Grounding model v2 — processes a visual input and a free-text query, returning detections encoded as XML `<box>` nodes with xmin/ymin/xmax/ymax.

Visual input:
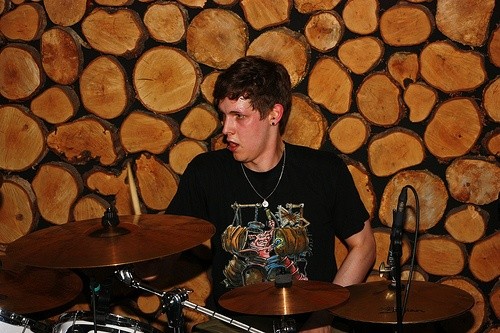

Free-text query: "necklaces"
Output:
<box><xmin>240</xmin><ymin>139</ymin><xmax>286</xmax><ymax>208</ymax></box>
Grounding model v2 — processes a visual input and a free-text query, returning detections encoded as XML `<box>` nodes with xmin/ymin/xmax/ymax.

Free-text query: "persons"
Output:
<box><xmin>165</xmin><ymin>56</ymin><xmax>377</xmax><ymax>333</ymax></box>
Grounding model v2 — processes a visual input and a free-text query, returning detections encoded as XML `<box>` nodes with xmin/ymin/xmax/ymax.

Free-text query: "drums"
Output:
<box><xmin>53</xmin><ymin>310</ymin><xmax>145</xmax><ymax>333</ymax></box>
<box><xmin>0</xmin><ymin>307</ymin><xmax>53</xmax><ymax>333</ymax></box>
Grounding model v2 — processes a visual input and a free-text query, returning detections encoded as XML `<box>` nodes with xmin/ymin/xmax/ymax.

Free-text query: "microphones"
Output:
<box><xmin>387</xmin><ymin>186</ymin><xmax>407</xmax><ymax>266</ymax></box>
<box><xmin>89</xmin><ymin>277</ymin><xmax>102</xmax><ymax>296</ymax></box>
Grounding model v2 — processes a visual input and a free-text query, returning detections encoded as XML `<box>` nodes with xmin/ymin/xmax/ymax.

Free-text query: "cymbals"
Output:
<box><xmin>328</xmin><ymin>280</ymin><xmax>475</xmax><ymax>324</ymax></box>
<box><xmin>5</xmin><ymin>215</ymin><xmax>217</xmax><ymax>269</ymax></box>
<box><xmin>0</xmin><ymin>255</ymin><xmax>83</xmax><ymax>315</ymax></box>
<box><xmin>219</xmin><ymin>280</ymin><xmax>350</xmax><ymax>315</ymax></box>
